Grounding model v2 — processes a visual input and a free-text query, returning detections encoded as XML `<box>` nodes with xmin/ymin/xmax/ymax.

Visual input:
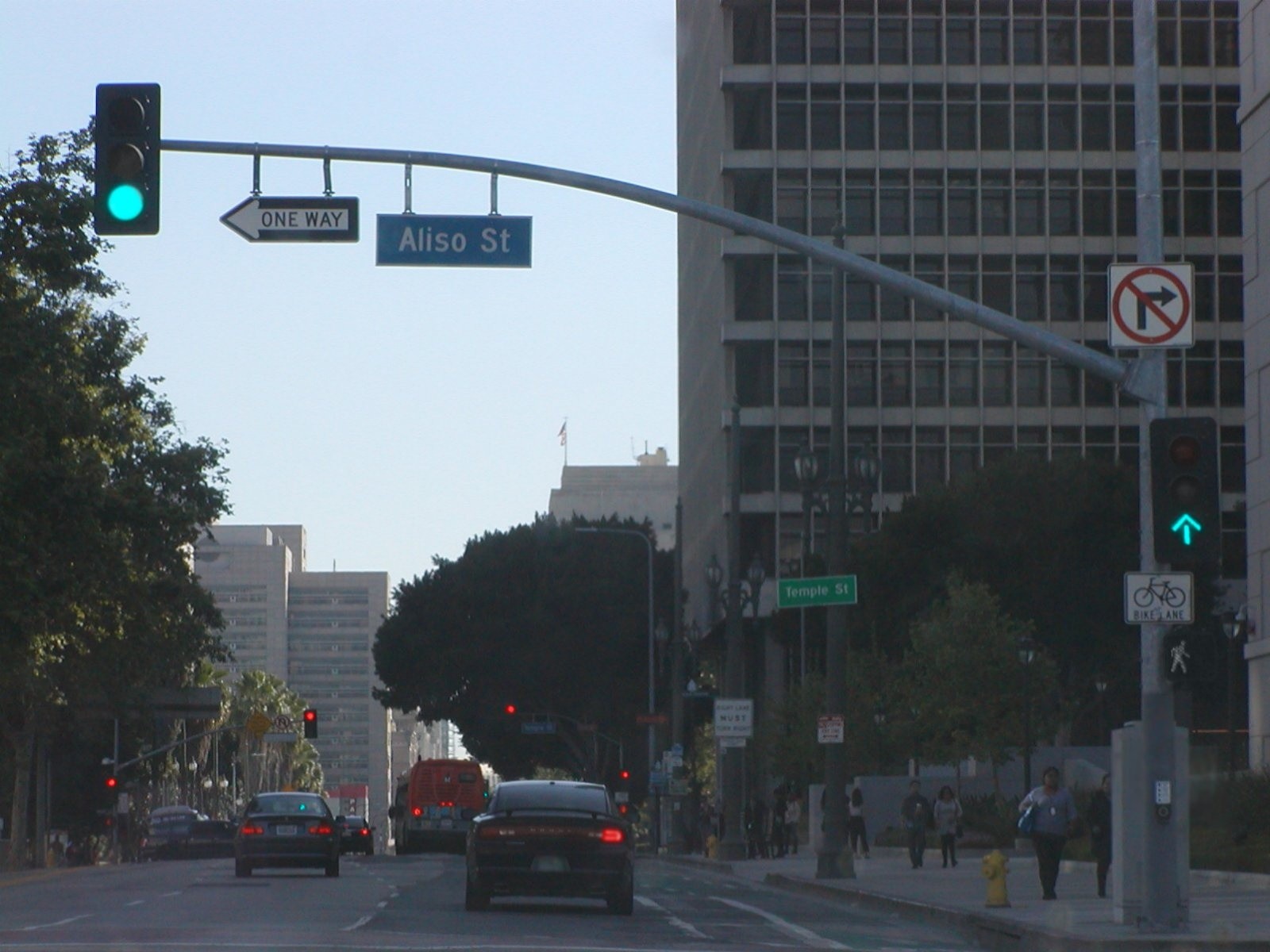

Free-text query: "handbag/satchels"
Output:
<box><xmin>1016</xmin><ymin>792</ymin><xmax>1040</xmax><ymax>833</ymax></box>
<box><xmin>956</xmin><ymin>823</ymin><xmax>963</xmax><ymax>838</ymax></box>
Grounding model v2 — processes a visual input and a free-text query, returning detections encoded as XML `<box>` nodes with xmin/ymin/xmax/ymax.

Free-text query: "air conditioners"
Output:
<box><xmin>331</xmin><ymin>622</ymin><xmax>338</xmax><ymax>627</ymax></box>
<box><xmin>332</xmin><ymin>758</ymin><xmax>339</xmax><ymax>764</ymax></box>
<box><xmin>332</xmin><ymin>645</ymin><xmax>338</xmax><ymax>650</ymax></box>
<box><xmin>331</xmin><ymin>715</ymin><xmax>339</xmax><ymax>721</ymax></box>
<box><xmin>229</xmin><ymin>667</ymin><xmax>236</xmax><ymax>673</ymax></box>
<box><xmin>229</xmin><ymin>619</ymin><xmax>236</xmax><ymax>626</ymax></box>
<box><xmin>230</xmin><ymin>643</ymin><xmax>237</xmax><ymax>649</ymax></box>
<box><xmin>331</xmin><ymin>692</ymin><xmax>338</xmax><ymax>698</ymax></box>
<box><xmin>229</xmin><ymin>690</ymin><xmax>237</xmax><ymax>696</ymax></box>
<box><xmin>332</xmin><ymin>598</ymin><xmax>338</xmax><ymax>603</ymax></box>
<box><xmin>331</xmin><ymin>785</ymin><xmax>339</xmax><ymax>791</ymax></box>
<box><xmin>331</xmin><ymin>738</ymin><xmax>338</xmax><ymax>743</ymax></box>
<box><xmin>230</xmin><ymin>596</ymin><xmax>236</xmax><ymax>602</ymax></box>
<box><xmin>332</xmin><ymin>668</ymin><xmax>338</xmax><ymax>674</ymax></box>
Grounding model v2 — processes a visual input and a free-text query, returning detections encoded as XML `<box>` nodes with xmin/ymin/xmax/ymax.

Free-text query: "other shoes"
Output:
<box><xmin>1042</xmin><ymin>890</ymin><xmax>1058</xmax><ymax>901</ymax></box>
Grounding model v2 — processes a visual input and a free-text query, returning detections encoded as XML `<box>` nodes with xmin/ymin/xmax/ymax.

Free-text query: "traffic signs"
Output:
<box><xmin>220</xmin><ymin>195</ymin><xmax>359</xmax><ymax>242</ymax></box>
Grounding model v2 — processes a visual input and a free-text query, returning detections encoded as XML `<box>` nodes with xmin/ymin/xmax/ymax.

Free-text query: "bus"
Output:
<box><xmin>388</xmin><ymin>755</ymin><xmax>490</xmax><ymax>857</ymax></box>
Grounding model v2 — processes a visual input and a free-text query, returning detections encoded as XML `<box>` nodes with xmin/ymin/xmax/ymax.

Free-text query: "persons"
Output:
<box><xmin>297</xmin><ymin>778</ymin><xmax>330</xmax><ymax>800</ymax></box>
<box><xmin>655</xmin><ymin>801</ymin><xmax>726</xmax><ymax>857</ymax></box>
<box><xmin>900</xmin><ymin>780</ymin><xmax>929</xmax><ymax>869</ymax></box>
<box><xmin>934</xmin><ymin>786</ymin><xmax>963</xmax><ymax>868</ymax></box>
<box><xmin>1089</xmin><ymin>773</ymin><xmax>1112</xmax><ymax>898</ymax></box>
<box><xmin>1019</xmin><ymin>766</ymin><xmax>1078</xmax><ymax>901</ymax></box>
<box><xmin>848</xmin><ymin>787</ymin><xmax>871</xmax><ymax>859</ymax></box>
<box><xmin>744</xmin><ymin>788</ymin><xmax>801</xmax><ymax>860</ymax></box>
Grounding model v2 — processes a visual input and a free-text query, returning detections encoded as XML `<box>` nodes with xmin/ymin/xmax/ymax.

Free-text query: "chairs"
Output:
<box><xmin>261</xmin><ymin>806</ymin><xmax>274</xmax><ymax>812</ymax></box>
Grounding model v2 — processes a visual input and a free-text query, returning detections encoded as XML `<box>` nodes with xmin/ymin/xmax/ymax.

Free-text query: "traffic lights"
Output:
<box><xmin>618</xmin><ymin>770</ymin><xmax>632</xmax><ymax>779</ymax></box>
<box><xmin>506</xmin><ymin>703</ymin><xmax>516</xmax><ymax>716</ymax></box>
<box><xmin>619</xmin><ymin>806</ymin><xmax>628</xmax><ymax>816</ymax></box>
<box><xmin>1164</xmin><ymin>626</ymin><xmax>1211</xmax><ymax>675</ymax></box>
<box><xmin>1149</xmin><ymin>419</ymin><xmax>1222</xmax><ymax>566</ymax></box>
<box><xmin>482</xmin><ymin>780</ymin><xmax>490</xmax><ymax>799</ymax></box>
<box><xmin>303</xmin><ymin>708</ymin><xmax>319</xmax><ymax>737</ymax></box>
<box><xmin>105</xmin><ymin>777</ymin><xmax>118</xmax><ymax>788</ymax></box>
<box><xmin>93</xmin><ymin>83</ymin><xmax>160</xmax><ymax>235</ymax></box>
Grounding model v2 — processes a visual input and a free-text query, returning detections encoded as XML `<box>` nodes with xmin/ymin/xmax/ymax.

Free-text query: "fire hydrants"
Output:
<box><xmin>705</xmin><ymin>834</ymin><xmax>721</xmax><ymax>860</ymax></box>
<box><xmin>981</xmin><ymin>850</ymin><xmax>1013</xmax><ymax>908</ymax></box>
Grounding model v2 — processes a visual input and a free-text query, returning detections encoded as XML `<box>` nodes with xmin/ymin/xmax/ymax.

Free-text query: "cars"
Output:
<box><xmin>235</xmin><ymin>791</ymin><xmax>341</xmax><ymax>878</ymax></box>
<box><xmin>146</xmin><ymin>804</ymin><xmax>241</xmax><ymax>857</ymax></box>
<box><xmin>336</xmin><ymin>815</ymin><xmax>378</xmax><ymax>856</ymax></box>
<box><xmin>466</xmin><ymin>781</ymin><xmax>635</xmax><ymax>917</ymax></box>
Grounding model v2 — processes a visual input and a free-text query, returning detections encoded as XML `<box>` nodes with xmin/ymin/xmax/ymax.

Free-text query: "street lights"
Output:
<box><xmin>104</xmin><ymin>757</ymin><xmax>121</xmax><ymax>865</ymax></box>
<box><xmin>574</xmin><ymin>526</ymin><xmax>660</xmax><ymax>856</ymax></box>
<box><xmin>203</xmin><ymin>773</ymin><xmax>229</xmax><ymax>822</ymax></box>
<box><xmin>702</xmin><ymin>391</ymin><xmax>766</xmax><ymax>864</ymax></box>
<box><xmin>173</xmin><ymin>756</ymin><xmax>197</xmax><ymax>805</ymax></box>
<box><xmin>796</xmin><ymin>203</ymin><xmax>878</xmax><ymax>883</ymax></box>
